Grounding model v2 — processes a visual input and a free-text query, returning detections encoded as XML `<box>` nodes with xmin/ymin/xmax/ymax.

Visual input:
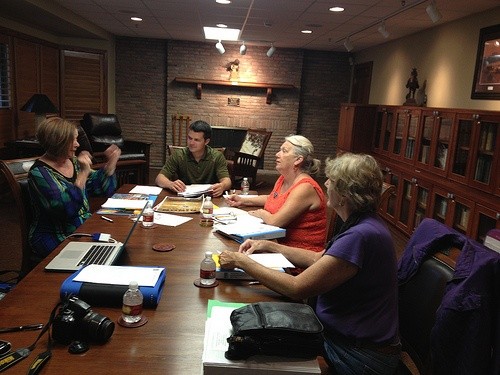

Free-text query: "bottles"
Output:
<box><xmin>200</xmin><ymin>251</ymin><xmax>216</xmax><ymax>286</ymax></box>
<box><xmin>142</xmin><ymin>203</ymin><xmax>154</xmax><ymax>227</ymax></box>
<box><xmin>203</xmin><ymin>197</ymin><xmax>213</xmax><ymax>218</ymax></box>
<box><xmin>241</xmin><ymin>178</ymin><xmax>250</xmax><ymax>195</ymax></box>
<box><xmin>121</xmin><ymin>281</ymin><xmax>144</xmax><ymax>323</ymax></box>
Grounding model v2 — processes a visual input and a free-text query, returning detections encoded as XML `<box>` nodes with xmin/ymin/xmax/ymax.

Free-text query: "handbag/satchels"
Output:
<box><xmin>225</xmin><ymin>301</ymin><xmax>324</xmax><ymax>361</ymax></box>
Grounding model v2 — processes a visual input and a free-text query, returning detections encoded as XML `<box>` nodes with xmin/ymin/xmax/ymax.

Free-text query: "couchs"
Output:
<box><xmin>81</xmin><ymin>114</ymin><xmax>152</xmax><ymax>186</ymax></box>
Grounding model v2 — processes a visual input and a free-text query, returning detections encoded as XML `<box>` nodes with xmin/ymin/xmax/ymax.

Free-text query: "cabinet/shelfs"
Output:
<box><xmin>337</xmin><ymin>102</ymin><xmax>500</xmax><ymax>250</ymax></box>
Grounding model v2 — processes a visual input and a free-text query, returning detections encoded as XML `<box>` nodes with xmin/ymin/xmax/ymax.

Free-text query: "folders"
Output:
<box><xmin>217</xmin><ymin>223</ymin><xmax>286</xmax><ymax>244</ymax></box>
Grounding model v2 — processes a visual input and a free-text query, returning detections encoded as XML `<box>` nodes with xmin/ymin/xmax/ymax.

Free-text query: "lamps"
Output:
<box><xmin>426</xmin><ymin>0</ymin><xmax>443</xmax><ymax>23</ymax></box>
<box><xmin>266</xmin><ymin>41</ymin><xmax>276</xmax><ymax>58</ymax></box>
<box><xmin>377</xmin><ymin>20</ymin><xmax>391</xmax><ymax>39</ymax></box>
<box><xmin>215</xmin><ymin>40</ymin><xmax>226</xmax><ymax>55</ymax></box>
<box><xmin>239</xmin><ymin>41</ymin><xmax>247</xmax><ymax>55</ymax></box>
<box><xmin>343</xmin><ymin>37</ymin><xmax>354</xmax><ymax>52</ymax></box>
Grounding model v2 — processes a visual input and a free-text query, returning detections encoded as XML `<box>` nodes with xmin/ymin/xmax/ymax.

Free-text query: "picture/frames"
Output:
<box><xmin>471</xmin><ymin>25</ymin><xmax>500</xmax><ymax>100</ymax></box>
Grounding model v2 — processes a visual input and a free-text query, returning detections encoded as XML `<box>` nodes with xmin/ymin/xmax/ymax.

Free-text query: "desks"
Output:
<box><xmin>0</xmin><ymin>183</ymin><xmax>322</xmax><ymax>375</ymax></box>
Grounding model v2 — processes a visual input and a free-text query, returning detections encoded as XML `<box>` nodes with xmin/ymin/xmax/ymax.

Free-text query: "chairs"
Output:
<box><xmin>223</xmin><ymin>129</ymin><xmax>272</xmax><ymax>188</ymax></box>
<box><xmin>0</xmin><ymin>155</ymin><xmax>41</xmax><ymax>283</ymax></box>
<box><xmin>168</xmin><ymin>145</ymin><xmax>227</xmax><ymax>156</ymax></box>
<box><xmin>392</xmin><ymin>218</ymin><xmax>500</xmax><ymax>375</ymax></box>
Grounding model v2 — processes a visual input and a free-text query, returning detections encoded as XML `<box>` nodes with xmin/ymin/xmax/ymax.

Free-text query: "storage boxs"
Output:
<box><xmin>202</xmin><ymin>298</ymin><xmax>321</xmax><ymax>375</ymax></box>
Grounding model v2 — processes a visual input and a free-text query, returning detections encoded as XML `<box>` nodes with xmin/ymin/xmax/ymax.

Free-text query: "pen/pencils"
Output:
<box><xmin>233</xmin><ymin>188</ymin><xmax>235</xmax><ymax>193</ymax></box>
<box><xmin>225</xmin><ymin>191</ymin><xmax>229</xmax><ymax>199</ymax></box>
<box><xmin>100</xmin><ymin>215</ymin><xmax>113</xmax><ymax>221</ymax></box>
<box><xmin>249</xmin><ymin>282</ymin><xmax>260</xmax><ymax>284</ymax></box>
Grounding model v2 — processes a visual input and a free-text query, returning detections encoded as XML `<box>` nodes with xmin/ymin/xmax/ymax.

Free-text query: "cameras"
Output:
<box><xmin>52</xmin><ymin>292</ymin><xmax>115</xmax><ymax>344</ymax></box>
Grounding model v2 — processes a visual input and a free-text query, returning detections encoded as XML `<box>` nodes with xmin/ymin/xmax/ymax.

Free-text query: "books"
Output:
<box><xmin>406</xmin><ymin>125</ymin><xmax>493</xmax><ymax>228</ymax></box>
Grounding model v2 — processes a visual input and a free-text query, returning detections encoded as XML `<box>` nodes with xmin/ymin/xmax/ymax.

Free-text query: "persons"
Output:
<box><xmin>226</xmin><ymin>135</ymin><xmax>327</xmax><ymax>275</ymax></box>
<box><xmin>27</xmin><ymin>117</ymin><xmax>121</xmax><ymax>256</ymax></box>
<box><xmin>218</xmin><ymin>151</ymin><xmax>403</xmax><ymax>375</ymax></box>
<box><xmin>406</xmin><ymin>68</ymin><xmax>419</xmax><ymax>98</ymax></box>
<box><xmin>155</xmin><ymin>120</ymin><xmax>231</xmax><ymax>197</ymax></box>
